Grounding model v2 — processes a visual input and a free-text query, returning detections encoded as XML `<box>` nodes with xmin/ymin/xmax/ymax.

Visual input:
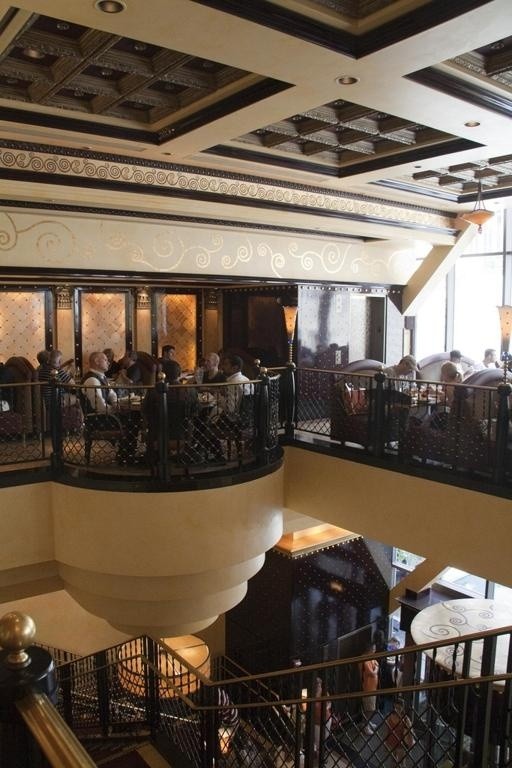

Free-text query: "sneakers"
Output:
<box><xmin>360</xmin><ymin>721</ymin><xmax>377</xmax><ymax>736</ymax></box>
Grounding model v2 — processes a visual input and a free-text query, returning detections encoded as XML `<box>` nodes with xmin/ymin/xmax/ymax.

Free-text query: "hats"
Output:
<box><xmin>403</xmin><ymin>355</ymin><xmax>421</xmax><ymax>374</ymax></box>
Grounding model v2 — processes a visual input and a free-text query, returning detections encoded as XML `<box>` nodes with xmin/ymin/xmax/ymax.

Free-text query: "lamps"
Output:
<box><xmin>282</xmin><ymin>305</ymin><xmax>302</xmax><ymax>362</ymax></box>
<box><xmin>461</xmin><ymin>164</ymin><xmax>495</xmax><ymax>235</ymax></box>
<box><xmin>495</xmin><ymin>304</ymin><xmax>511</xmax><ymax>384</ymax></box>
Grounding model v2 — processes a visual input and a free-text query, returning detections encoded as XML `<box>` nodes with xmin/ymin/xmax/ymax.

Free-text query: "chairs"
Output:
<box><xmin>0</xmin><ymin>343</ymin><xmax>511</xmax><ymax>497</ymax></box>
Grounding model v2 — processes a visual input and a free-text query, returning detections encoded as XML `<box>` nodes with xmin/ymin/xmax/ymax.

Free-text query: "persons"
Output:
<box><xmin>470</xmin><ymin>349</ymin><xmax>499</xmax><ymax>375</ymax></box>
<box><xmin>372</xmin><ymin>355</ymin><xmax>421</xmax><ymax>399</ymax></box>
<box><xmin>313</xmin><ymin>677</ymin><xmax>333</xmax><ymax>768</ymax></box>
<box><xmin>387</xmin><ymin>636</ymin><xmax>401</xmax><ymax>651</ymax></box>
<box><xmin>1</xmin><ymin>360</ymin><xmax>19</xmax><ymax>415</ymax></box>
<box><xmin>38</xmin><ymin>343</ymin><xmax>276</xmax><ymax>468</ymax></box>
<box><xmin>423</xmin><ymin>361</ymin><xmax>463</xmax><ymax>433</ymax></box>
<box><xmin>384</xmin><ymin>698</ymin><xmax>414</xmax><ymax>768</ymax></box>
<box><xmin>216</xmin><ymin>688</ymin><xmax>239</xmax><ymax>754</ymax></box>
<box><xmin>357</xmin><ymin>641</ymin><xmax>380</xmax><ymax>737</ymax></box>
<box><xmin>443</xmin><ymin>351</ymin><xmax>473</xmax><ymax>382</ymax></box>
